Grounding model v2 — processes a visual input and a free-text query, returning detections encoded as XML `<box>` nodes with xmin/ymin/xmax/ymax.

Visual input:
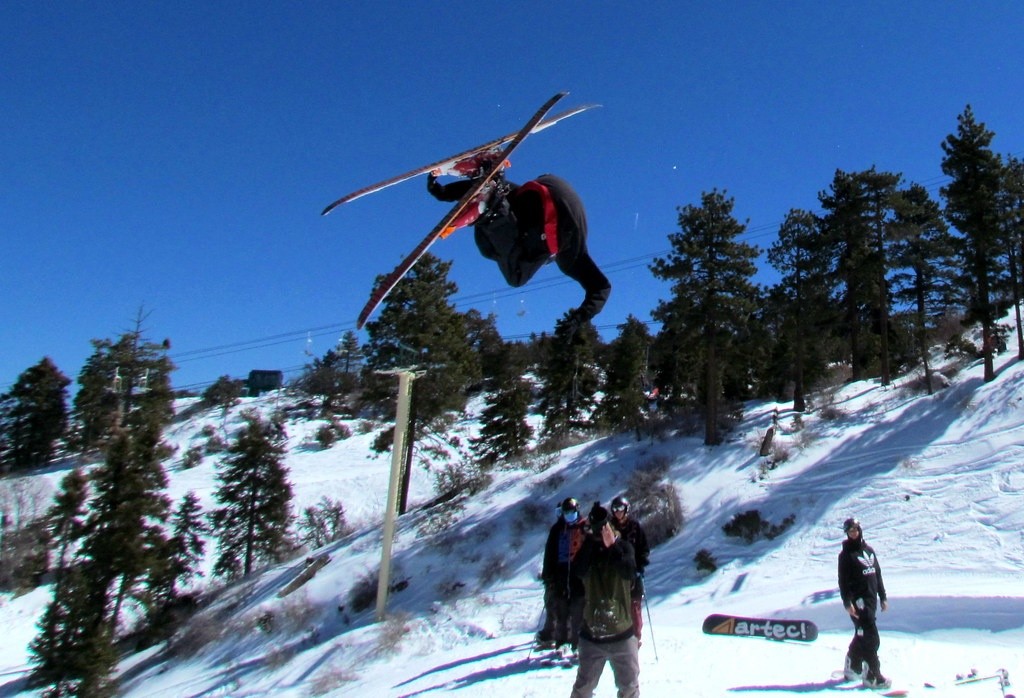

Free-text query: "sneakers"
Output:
<box><xmin>862</xmin><ymin>671</ymin><xmax>892</xmax><ymax>689</ymax></box>
<box><xmin>432</xmin><ymin>145</ymin><xmax>511</xmax><ymax>178</ymax></box>
<box><xmin>844</xmin><ymin>661</ymin><xmax>864</xmax><ymax>681</ymax></box>
<box><xmin>440</xmin><ymin>179</ymin><xmax>510</xmax><ymax>241</ymax></box>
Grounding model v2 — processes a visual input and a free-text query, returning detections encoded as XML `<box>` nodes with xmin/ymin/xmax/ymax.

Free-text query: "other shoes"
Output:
<box><xmin>536</xmin><ymin>629</ymin><xmax>554</xmax><ymax>645</ymax></box>
<box><xmin>557</xmin><ymin>648</ymin><xmax>578</xmax><ymax>658</ymax></box>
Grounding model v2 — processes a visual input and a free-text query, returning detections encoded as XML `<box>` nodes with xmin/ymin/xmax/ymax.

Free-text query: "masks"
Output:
<box><xmin>563</xmin><ymin>512</ymin><xmax>578</xmax><ymax>522</ymax></box>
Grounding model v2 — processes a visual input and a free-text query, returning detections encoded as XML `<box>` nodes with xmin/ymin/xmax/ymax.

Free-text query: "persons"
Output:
<box><xmin>537</xmin><ymin>497</ymin><xmax>584</xmax><ymax>656</ymax></box>
<box><xmin>428</xmin><ymin>172</ymin><xmax>610</xmax><ymax>337</ymax></box>
<box><xmin>570</xmin><ymin>501</ymin><xmax>640</xmax><ymax>698</ymax></box>
<box><xmin>611</xmin><ymin>496</ymin><xmax>650</xmax><ymax>646</ymax></box>
<box><xmin>838</xmin><ymin>518</ymin><xmax>892</xmax><ymax>690</ymax></box>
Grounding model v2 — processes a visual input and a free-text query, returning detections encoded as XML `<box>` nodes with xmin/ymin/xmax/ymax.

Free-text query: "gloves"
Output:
<box><xmin>427</xmin><ymin>173</ymin><xmax>437</xmax><ymax>192</ymax></box>
<box><xmin>555</xmin><ymin>317</ymin><xmax>580</xmax><ymax>343</ymax></box>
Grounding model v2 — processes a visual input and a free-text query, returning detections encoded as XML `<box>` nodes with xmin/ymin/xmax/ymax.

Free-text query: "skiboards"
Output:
<box><xmin>542</xmin><ymin>647</ymin><xmax>580</xmax><ymax>668</ymax></box>
<box><xmin>320</xmin><ymin>88</ymin><xmax>605</xmax><ymax>329</ymax></box>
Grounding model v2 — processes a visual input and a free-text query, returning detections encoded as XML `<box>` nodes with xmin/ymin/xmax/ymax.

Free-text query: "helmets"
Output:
<box><xmin>843</xmin><ymin>518</ymin><xmax>861</xmax><ymax>532</ymax></box>
<box><xmin>612</xmin><ymin>497</ymin><xmax>631</xmax><ymax>515</ymax></box>
<box><xmin>561</xmin><ymin>497</ymin><xmax>579</xmax><ymax>510</ymax></box>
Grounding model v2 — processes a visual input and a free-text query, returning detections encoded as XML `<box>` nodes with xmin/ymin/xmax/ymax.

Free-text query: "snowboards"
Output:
<box><xmin>701</xmin><ymin>612</ymin><xmax>819</xmax><ymax>643</ymax></box>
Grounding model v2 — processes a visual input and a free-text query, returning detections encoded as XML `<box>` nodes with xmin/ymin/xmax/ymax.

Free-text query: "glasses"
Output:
<box><xmin>611</xmin><ymin>505</ymin><xmax>627</xmax><ymax>512</ymax></box>
<box><xmin>563</xmin><ymin>507</ymin><xmax>577</xmax><ymax>514</ymax></box>
